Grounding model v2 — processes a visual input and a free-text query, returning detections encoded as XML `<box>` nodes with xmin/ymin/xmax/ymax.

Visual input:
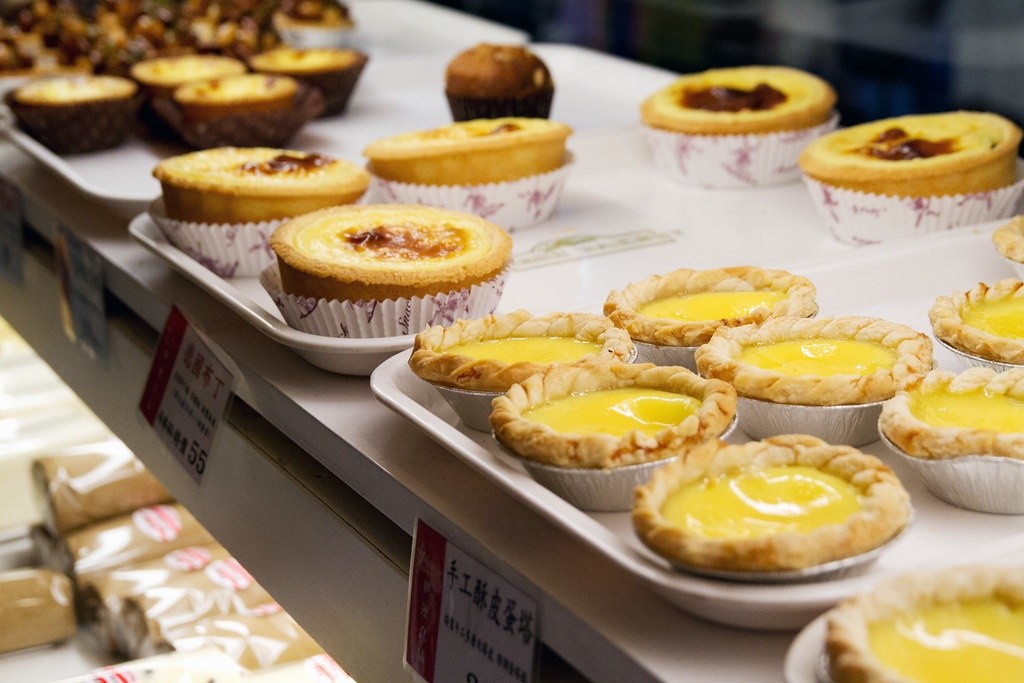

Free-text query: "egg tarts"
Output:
<box><xmin>406</xmin><ymin>217</ymin><xmax>1024</xmax><ymax>683</ymax></box>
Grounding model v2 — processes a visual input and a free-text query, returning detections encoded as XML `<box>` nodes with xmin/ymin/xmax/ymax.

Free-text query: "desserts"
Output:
<box><xmin>0</xmin><ymin>0</ymin><xmax>1024</xmax><ymax>338</ymax></box>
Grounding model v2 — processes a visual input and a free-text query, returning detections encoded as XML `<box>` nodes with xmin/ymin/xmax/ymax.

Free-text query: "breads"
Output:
<box><xmin>0</xmin><ymin>433</ymin><xmax>355</xmax><ymax>683</ymax></box>
